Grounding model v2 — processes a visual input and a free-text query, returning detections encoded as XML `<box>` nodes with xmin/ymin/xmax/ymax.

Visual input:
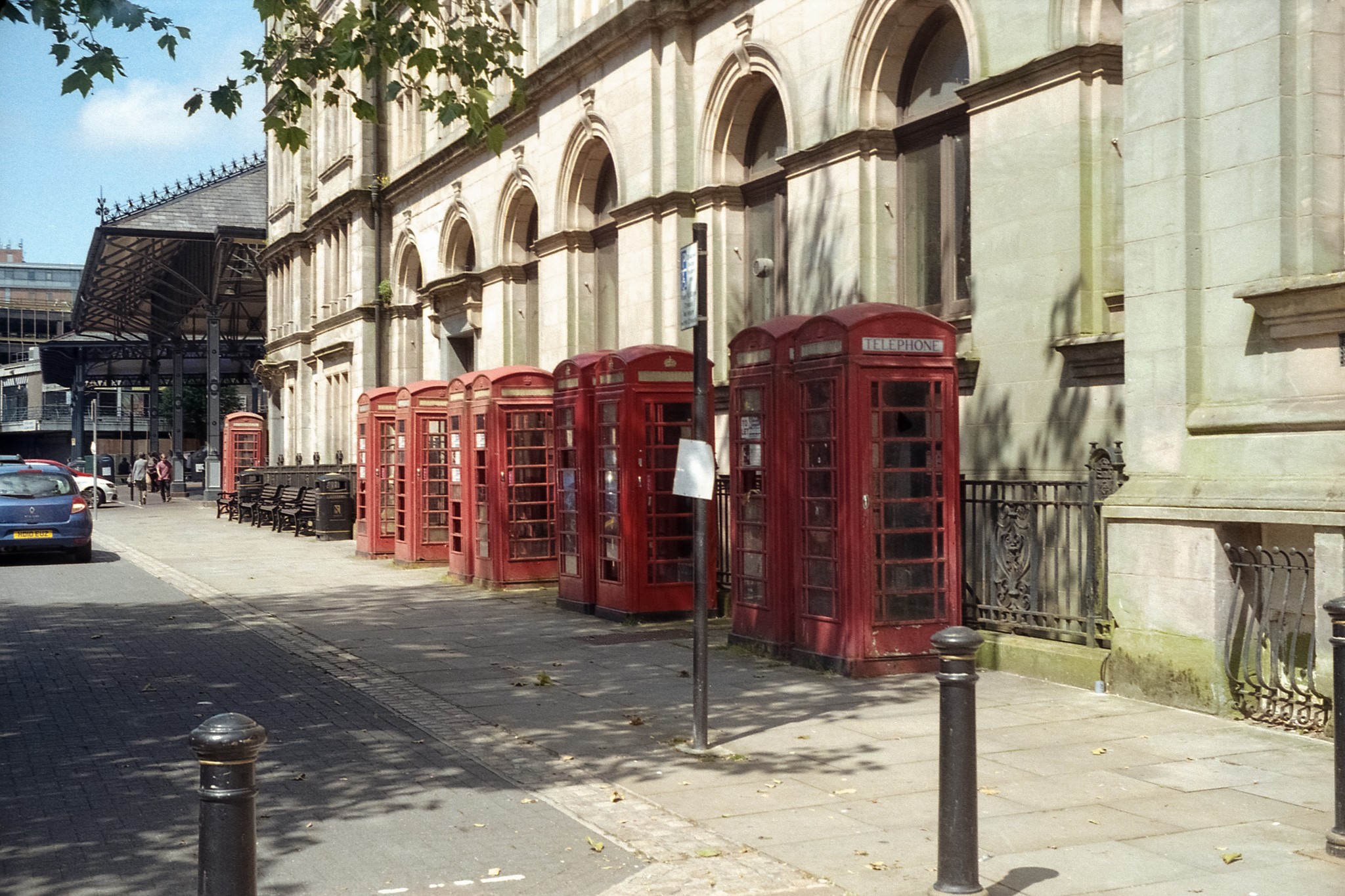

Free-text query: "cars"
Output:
<box><xmin>0</xmin><ymin>465</ymin><xmax>92</xmax><ymax>563</ymax></box>
<box><xmin>0</xmin><ymin>454</ymin><xmax>119</xmax><ymax>509</ymax></box>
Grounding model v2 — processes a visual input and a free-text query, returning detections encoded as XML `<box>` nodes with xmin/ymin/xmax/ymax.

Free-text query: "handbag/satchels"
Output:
<box><xmin>126</xmin><ymin>473</ymin><xmax>132</xmax><ymax>483</ymax></box>
<box><xmin>145</xmin><ymin>473</ymin><xmax>152</xmax><ymax>484</ymax></box>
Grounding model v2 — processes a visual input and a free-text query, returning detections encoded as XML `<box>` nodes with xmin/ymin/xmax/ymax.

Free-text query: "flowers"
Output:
<box><xmin>374</xmin><ymin>171</ymin><xmax>392</xmax><ymax>190</ymax></box>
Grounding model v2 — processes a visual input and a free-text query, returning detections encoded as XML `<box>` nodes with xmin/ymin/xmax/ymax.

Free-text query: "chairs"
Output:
<box><xmin>215</xmin><ymin>481</ymin><xmax>320</xmax><ymax>539</ymax></box>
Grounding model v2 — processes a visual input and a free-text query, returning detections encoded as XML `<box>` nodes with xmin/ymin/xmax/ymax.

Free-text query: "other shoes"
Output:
<box><xmin>142</xmin><ymin>496</ymin><xmax>146</xmax><ymax>504</ymax></box>
<box><xmin>166</xmin><ymin>496</ymin><xmax>171</xmax><ymax>502</ymax></box>
<box><xmin>138</xmin><ymin>502</ymin><xmax>143</xmax><ymax>506</ymax></box>
<box><xmin>162</xmin><ymin>499</ymin><xmax>165</xmax><ymax>503</ymax></box>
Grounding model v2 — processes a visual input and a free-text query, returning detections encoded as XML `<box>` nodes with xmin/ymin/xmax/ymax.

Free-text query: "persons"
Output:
<box><xmin>131</xmin><ymin>451</ymin><xmax>148</xmax><ymax>505</ymax></box>
<box><xmin>118</xmin><ymin>457</ymin><xmax>131</xmax><ymax>484</ymax></box>
<box><xmin>166</xmin><ymin>450</ymin><xmax>173</xmax><ymax>464</ymax></box>
<box><xmin>155</xmin><ymin>454</ymin><xmax>173</xmax><ymax>502</ymax></box>
<box><xmin>146</xmin><ymin>453</ymin><xmax>160</xmax><ymax>493</ymax></box>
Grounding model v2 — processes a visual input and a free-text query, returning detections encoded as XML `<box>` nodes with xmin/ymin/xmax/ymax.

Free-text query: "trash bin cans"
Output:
<box><xmin>238</xmin><ymin>469</ymin><xmax>263</xmax><ymax>518</ymax></box>
<box><xmin>314</xmin><ymin>472</ymin><xmax>352</xmax><ymax>541</ymax></box>
